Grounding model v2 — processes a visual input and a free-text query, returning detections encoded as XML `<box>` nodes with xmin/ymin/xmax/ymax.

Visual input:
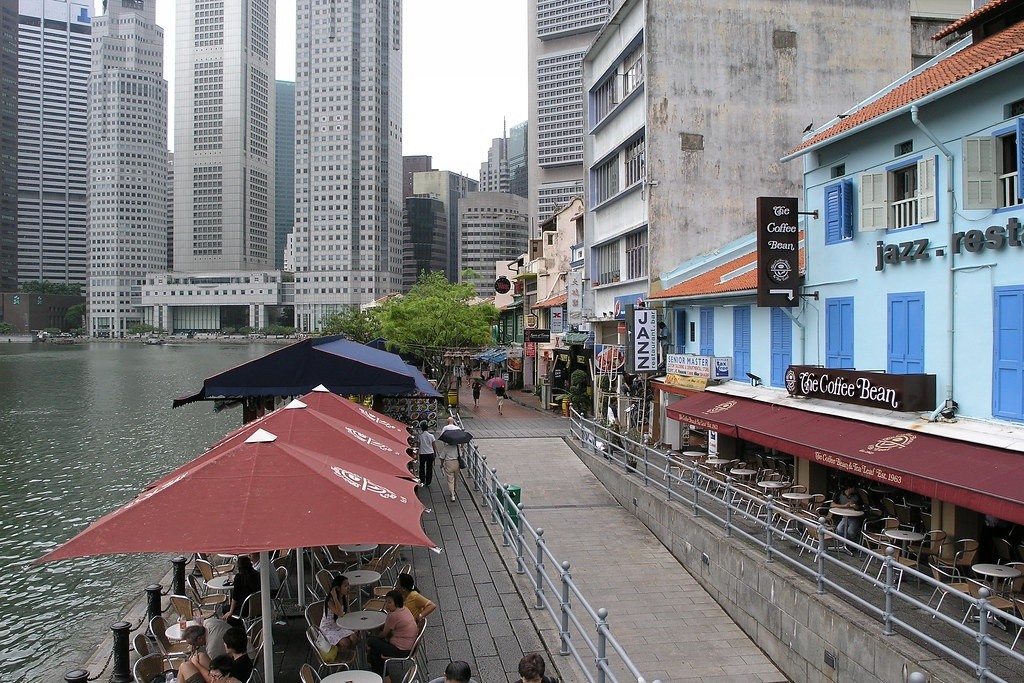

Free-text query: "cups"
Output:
<box><xmin>180</xmin><ymin>620</ymin><xmax>186</xmax><ymax>631</ymax></box>
<box><xmin>166</xmin><ymin>671</ymin><xmax>174</xmax><ymax>683</ymax></box>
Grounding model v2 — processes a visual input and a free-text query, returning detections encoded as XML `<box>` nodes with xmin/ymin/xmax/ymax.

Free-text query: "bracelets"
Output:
<box><xmin>419</xmin><ymin>614</ymin><xmax>422</xmax><ymax>617</ymax></box>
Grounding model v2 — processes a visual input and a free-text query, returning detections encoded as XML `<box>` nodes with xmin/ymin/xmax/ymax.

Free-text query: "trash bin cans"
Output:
<box><xmin>497</xmin><ymin>485</ymin><xmax>521</xmax><ymax>529</ymax></box>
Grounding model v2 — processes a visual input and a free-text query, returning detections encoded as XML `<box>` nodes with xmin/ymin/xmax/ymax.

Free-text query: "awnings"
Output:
<box><xmin>668</xmin><ymin>388</ymin><xmax>1024</xmax><ymax>526</ymax></box>
<box><xmin>473</xmin><ymin>348</ymin><xmax>506</xmax><ymax>366</ymax></box>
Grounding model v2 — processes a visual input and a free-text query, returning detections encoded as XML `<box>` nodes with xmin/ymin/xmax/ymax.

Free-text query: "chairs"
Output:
<box><xmin>663</xmin><ymin>450</ymin><xmax>1024</xmax><ymax>651</ymax></box>
<box><xmin>298</xmin><ymin>543</ymin><xmax>428</xmax><ymax>683</ymax></box>
<box><xmin>132</xmin><ymin>544</ymin><xmax>292</xmax><ymax>683</ymax></box>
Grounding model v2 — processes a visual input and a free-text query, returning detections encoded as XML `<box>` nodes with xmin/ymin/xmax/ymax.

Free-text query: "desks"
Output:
<box><xmin>320</xmin><ymin>670</ymin><xmax>383</xmax><ymax>683</ymax></box>
<box><xmin>217</xmin><ymin>553</ymin><xmax>250</xmax><ymax>573</ymax></box>
<box><xmin>705</xmin><ymin>459</ymin><xmax>730</xmax><ymax>492</ymax></box>
<box><xmin>758</xmin><ymin>481</ymin><xmax>785</xmax><ymax>521</ymax></box>
<box><xmin>165</xmin><ymin>621</ymin><xmax>199</xmax><ymax>655</ymax></box>
<box><xmin>781</xmin><ymin>493</ymin><xmax>813</xmax><ymax>536</ymax></box>
<box><xmin>342</xmin><ymin>570</ymin><xmax>381</xmax><ymax>611</ymax></box>
<box><xmin>730</xmin><ymin>468</ymin><xmax>757</xmax><ymax>503</ymax></box>
<box><xmin>683</xmin><ymin>451</ymin><xmax>706</xmax><ymax>483</ymax></box>
<box><xmin>829</xmin><ymin>507</ymin><xmax>865</xmax><ymax>556</ymax></box>
<box><xmin>884</xmin><ymin>530</ymin><xmax>924</xmax><ymax>583</ymax></box>
<box><xmin>336</xmin><ymin>610</ymin><xmax>387</xmax><ymax>670</ymax></box>
<box><xmin>338</xmin><ymin>544</ymin><xmax>378</xmax><ymax>597</ymax></box>
<box><xmin>969</xmin><ymin>563</ymin><xmax>1021</xmax><ymax>631</ymax></box>
<box><xmin>206</xmin><ymin>575</ymin><xmax>236</xmax><ymax>605</ymax></box>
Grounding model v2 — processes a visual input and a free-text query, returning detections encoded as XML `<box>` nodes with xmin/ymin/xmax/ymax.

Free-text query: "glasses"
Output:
<box><xmin>208</xmin><ymin>674</ymin><xmax>223</xmax><ymax>680</ymax></box>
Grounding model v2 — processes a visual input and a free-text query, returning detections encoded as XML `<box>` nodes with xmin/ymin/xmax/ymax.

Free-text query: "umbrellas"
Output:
<box><xmin>35</xmin><ymin>428</ymin><xmax>438</xmax><ymax>683</ymax></box>
<box><xmin>208</xmin><ymin>382</ymin><xmax>416</xmax><ymax>448</ymax></box>
<box><xmin>438</xmin><ymin>430</ymin><xmax>473</xmax><ymax>445</ymax></box>
<box><xmin>488</xmin><ymin>376</ymin><xmax>505</xmax><ymax>389</ymax></box>
<box><xmin>138</xmin><ymin>397</ymin><xmax>418</xmax><ymax>607</ymax></box>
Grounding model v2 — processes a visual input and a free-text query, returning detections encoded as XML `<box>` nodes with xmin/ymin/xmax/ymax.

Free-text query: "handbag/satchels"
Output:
<box><xmin>458</xmin><ymin>456</ymin><xmax>467</xmax><ymax>469</ymax></box>
<box><xmin>311</xmin><ymin>626</ymin><xmax>338</xmax><ymax>662</ymax></box>
<box><xmin>503</xmin><ymin>394</ymin><xmax>508</xmax><ymax>399</ymax></box>
<box><xmin>147</xmin><ymin>669</ymin><xmax>178</xmax><ymax>683</ymax></box>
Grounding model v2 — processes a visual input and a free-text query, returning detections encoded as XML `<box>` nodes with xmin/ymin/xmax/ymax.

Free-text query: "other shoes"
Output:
<box><xmin>451</xmin><ymin>496</ymin><xmax>455</xmax><ymax>502</ymax></box>
<box><xmin>426</xmin><ymin>484</ymin><xmax>431</xmax><ymax>487</ymax></box>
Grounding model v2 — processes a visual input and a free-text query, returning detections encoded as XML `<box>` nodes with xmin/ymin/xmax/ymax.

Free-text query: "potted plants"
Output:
<box><xmin>625</xmin><ymin>428</ymin><xmax>640</xmax><ymax>472</ymax></box>
<box><xmin>567</xmin><ymin>369</ymin><xmax>590</xmax><ymax>418</ymax></box>
<box><xmin>600</xmin><ymin>418</ymin><xmax>616</xmax><ymax>459</ymax></box>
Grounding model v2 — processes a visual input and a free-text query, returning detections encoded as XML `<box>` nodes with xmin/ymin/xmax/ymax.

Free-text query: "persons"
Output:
<box><xmin>439</xmin><ymin>443</ymin><xmax>461</xmax><ymax>501</ymax></box>
<box><xmin>174</xmin><ymin>553</ymin><xmax>280</xmax><ymax>683</ymax></box>
<box><xmin>366</xmin><ymin>591</ymin><xmax>418</xmax><ymax>683</ymax></box>
<box><xmin>496</xmin><ymin>385</ymin><xmax>505</xmax><ymax>415</ymax></box>
<box><xmin>396</xmin><ymin>573</ymin><xmax>436</xmax><ymax>623</ymax></box>
<box><xmin>430</xmin><ymin>661</ymin><xmax>476</xmax><ymax>683</ymax></box>
<box><xmin>513</xmin><ymin>653</ymin><xmax>558</xmax><ymax>683</ymax></box>
<box><xmin>317</xmin><ymin>575</ymin><xmax>359</xmax><ymax>649</ymax></box>
<box><xmin>441</xmin><ymin>417</ymin><xmax>462</xmax><ymax>434</ymax></box>
<box><xmin>457</xmin><ymin>362</ymin><xmax>471</xmax><ymax>387</ymax></box>
<box><xmin>830</xmin><ymin>480</ymin><xmax>863</xmax><ymax>551</ymax></box>
<box><xmin>472</xmin><ymin>379</ymin><xmax>482</xmax><ymax>407</ymax></box>
<box><xmin>418</xmin><ymin>423</ymin><xmax>439</xmax><ymax>486</ymax></box>
